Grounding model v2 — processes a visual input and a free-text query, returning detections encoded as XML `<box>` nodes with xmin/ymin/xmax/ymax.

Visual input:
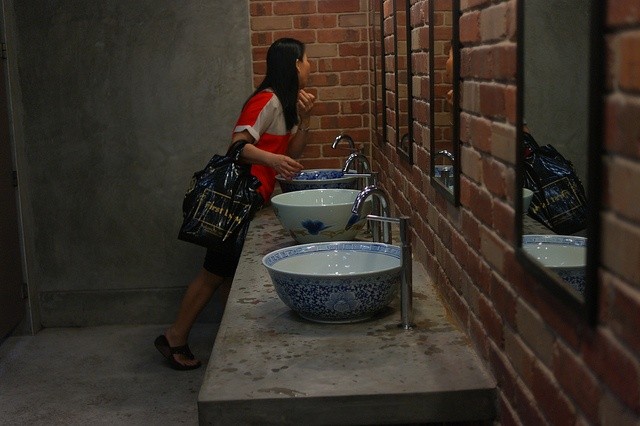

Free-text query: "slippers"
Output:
<box><xmin>154</xmin><ymin>335</ymin><xmax>201</xmax><ymax>371</ymax></box>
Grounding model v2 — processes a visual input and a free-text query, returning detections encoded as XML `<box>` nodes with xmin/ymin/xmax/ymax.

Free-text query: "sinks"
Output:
<box><xmin>434</xmin><ymin>164</ymin><xmax>454</xmax><ymax>179</ymax></box>
<box><xmin>272</xmin><ymin>188</ymin><xmax>372</xmax><ymax>242</ymax></box>
<box><xmin>263</xmin><ymin>241</ymin><xmax>413</xmax><ymax>323</ymax></box>
<box><xmin>276</xmin><ymin>168</ymin><xmax>358</xmax><ymax>192</ymax></box>
<box><xmin>523</xmin><ymin>187</ymin><xmax>533</xmax><ymax>199</ymax></box>
<box><xmin>522</xmin><ymin>234</ymin><xmax>587</xmax><ymax>267</ymax></box>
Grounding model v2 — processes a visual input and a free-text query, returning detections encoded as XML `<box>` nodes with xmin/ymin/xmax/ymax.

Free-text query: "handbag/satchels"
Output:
<box><xmin>176</xmin><ymin>138</ymin><xmax>264</xmax><ymax>250</ymax></box>
<box><xmin>520</xmin><ymin>132</ymin><xmax>587</xmax><ymax>235</ymax></box>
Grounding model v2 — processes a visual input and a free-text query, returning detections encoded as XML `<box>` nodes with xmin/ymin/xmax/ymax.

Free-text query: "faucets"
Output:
<box><xmin>350</xmin><ymin>184</ymin><xmax>393</xmax><ymax>244</ymax></box>
<box><xmin>343</xmin><ymin>151</ymin><xmax>371</xmax><ymax>188</ymax></box>
<box><xmin>433</xmin><ymin>149</ymin><xmax>454</xmax><ymax>163</ymax></box>
<box><xmin>331</xmin><ymin>132</ymin><xmax>356</xmax><ymax>170</ymax></box>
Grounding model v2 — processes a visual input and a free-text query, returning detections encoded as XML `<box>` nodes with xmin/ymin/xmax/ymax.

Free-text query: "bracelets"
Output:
<box><xmin>449</xmin><ymin>120</ymin><xmax>454</xmax><ymax>127</ymax></box>
<box><xmin>298</xmin><ymin>126</ymin><xmax>309</xmax><ymax>134</ymax></box>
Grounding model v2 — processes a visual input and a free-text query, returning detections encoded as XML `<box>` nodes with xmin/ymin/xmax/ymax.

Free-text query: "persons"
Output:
<box><xmin>445</xmin><ymin>40</ymin><xmax>454</xmax><ymax>132</ymax></box>
<box><xmin>523</xmin><ymin>119</ymin><xmax>534</xmax><ymax>160</ymax></box>
<box><xmin>154</xmin><ymin>37</ymin><xmax>316</xmax><ymax>370</ymax></box>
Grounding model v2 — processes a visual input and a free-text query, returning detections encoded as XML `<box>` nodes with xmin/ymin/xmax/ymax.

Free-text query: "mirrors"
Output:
<box><xmin>372</xmin><ymin>0</ymin><xmax>386</xmax><ymax>141</ymax></box>
<box><xmin>393</xmin><ymin>0</ymin><xmax>413</xmax><ymax>165</ymax></box>
<box><xmin>515</xmin><ymin>0</ymin><xmax>606</xmax><ymax>326</ymax></box>
<box><xmin>429</xmin><ymin>0</ymin><xmax>460</xmax><ymax>209</ymax></box>
